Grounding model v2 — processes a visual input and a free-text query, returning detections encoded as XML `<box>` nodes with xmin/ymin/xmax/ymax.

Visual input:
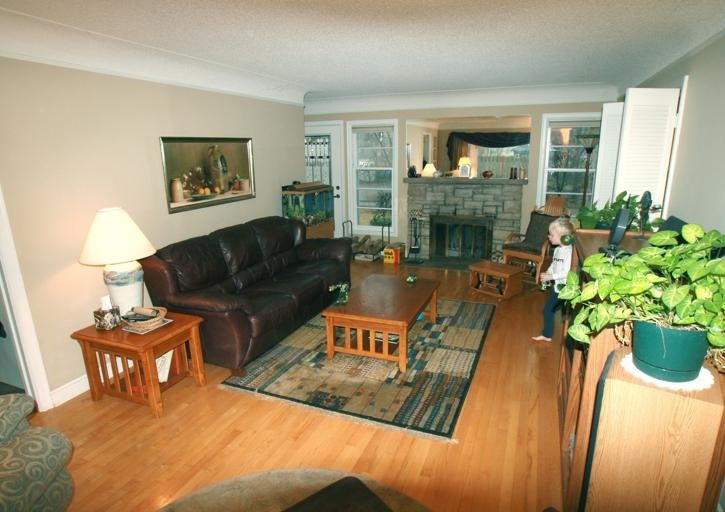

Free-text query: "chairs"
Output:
<box><xmin>0</xmin><ymin>387</ymin><xmax>76</xmax><ymax>512</ymax></box>
<box><xmin>501</xmin><ymin>195</ymin><xmax>578</xmax><ymax>285</ymax></box>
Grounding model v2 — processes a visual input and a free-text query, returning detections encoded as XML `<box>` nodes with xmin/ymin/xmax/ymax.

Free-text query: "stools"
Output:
<box><xmin>468</xmin><ymin>260</ymin><xmax>525</xmax><ymax>299</ymax></box>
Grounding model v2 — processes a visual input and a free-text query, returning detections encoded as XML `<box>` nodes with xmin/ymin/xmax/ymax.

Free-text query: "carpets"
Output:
<box><xmin>158</xmin><ymin>468</ymin><xmax>434</xmax><ymax>512</ymax></box>
<box><xmin>218</xmin><ymin>277</ymin><xmax>499</xmax><ymax>444</ymax></box>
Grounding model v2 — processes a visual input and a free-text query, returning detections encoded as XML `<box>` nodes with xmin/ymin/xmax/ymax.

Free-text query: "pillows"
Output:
<box><xmin>523</xmin><ymin>209</ymin><xmax>570</xmax><ymax>249</ymax></box>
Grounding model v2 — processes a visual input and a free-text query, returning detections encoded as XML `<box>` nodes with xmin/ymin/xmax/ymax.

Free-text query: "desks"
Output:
<box><xmin>70</xmin><ymin>310</ymin><xmax>210</xmax><ymax>408</ymax></box>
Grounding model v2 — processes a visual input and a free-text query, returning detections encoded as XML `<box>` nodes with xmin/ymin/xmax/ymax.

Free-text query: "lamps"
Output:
<box><xmin>78</xmin><ymin>204</ymin><xmax>158</xmax><ymax>317</ymax></box>
<box><xmin>573</xmin><ymin>131</ymin><xmax>602</xmax><ymax>208</ymax></box>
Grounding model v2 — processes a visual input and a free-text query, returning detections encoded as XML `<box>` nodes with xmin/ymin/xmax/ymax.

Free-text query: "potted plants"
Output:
<box><xmin>551</xmin><ymin>221</ymin><xmax>725</xmax><ymax>382</ymax></box>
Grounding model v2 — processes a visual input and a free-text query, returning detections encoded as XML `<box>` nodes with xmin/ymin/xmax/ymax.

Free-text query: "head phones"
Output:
<box><xmin>561</xmin><ymin>235</ymin><xmax>575</xmax><ymax>245</ymax></box>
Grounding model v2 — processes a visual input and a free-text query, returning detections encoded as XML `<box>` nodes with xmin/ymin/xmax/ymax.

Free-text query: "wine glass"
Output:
<box><xmin>379</xmin><ymin>132</ymin><xmax>384</xmax><ymax>148</ymax></box>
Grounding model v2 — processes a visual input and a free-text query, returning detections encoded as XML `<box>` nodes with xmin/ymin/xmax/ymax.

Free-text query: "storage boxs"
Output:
<box><xmin>384</xmin><ymin>246</ymin><xmax>405</xmax><ymax>265</ymax></box>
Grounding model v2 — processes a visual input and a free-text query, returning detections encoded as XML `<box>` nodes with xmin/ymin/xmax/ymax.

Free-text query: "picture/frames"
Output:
<box><xmin>159</xmin><ymin>135</ymin><xmax>256</xmax><ymax>214</ymax></box>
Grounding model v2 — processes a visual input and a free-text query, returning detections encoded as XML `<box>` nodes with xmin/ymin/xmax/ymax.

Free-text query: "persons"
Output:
<box><xmin>530</xmin><ymin>216</ymin><xmax>575</xmax><ymax>342</ymax></box>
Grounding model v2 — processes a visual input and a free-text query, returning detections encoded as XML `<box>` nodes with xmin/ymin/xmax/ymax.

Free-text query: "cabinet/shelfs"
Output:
<box><xmin>543</xmin><ymin>116</ymin><xmax>601</xmax><ymax>208</ymax></box>
<box><xmin>346</xmin><ymin>119</ymin><xmax>399</xmax><ymax>238</ymax></box>
<box><xmin>557</xmin><ymin>231</ymin><xmax>724</xmax><ymax>511</ymax></box>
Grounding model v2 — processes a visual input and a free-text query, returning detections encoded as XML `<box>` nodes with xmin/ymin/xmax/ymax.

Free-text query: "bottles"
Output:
<box><xmin>578</xmin><ymin>156</ymin><xmax>593</xmax><ymax>168</ymax></box>
<box><xmin>170</xmin><ymin>178</ymin><xmax>184</xmax><ymax>203</ymax></box>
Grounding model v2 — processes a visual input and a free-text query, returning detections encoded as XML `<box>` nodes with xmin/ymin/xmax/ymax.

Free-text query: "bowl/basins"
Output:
<box><xmin>121</xmin><ymin>306</ymin><xmax>167</xmax><ymax>329</ymax></box>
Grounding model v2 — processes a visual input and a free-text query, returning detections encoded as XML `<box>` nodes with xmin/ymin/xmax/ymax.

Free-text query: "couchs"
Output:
<box><xmin>139</xmin><ymin>213</ymin><xmax>354</xmax><ymax>375</ymax></box>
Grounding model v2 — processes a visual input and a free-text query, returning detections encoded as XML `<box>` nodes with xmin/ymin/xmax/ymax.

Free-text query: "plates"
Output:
<box><xmin>121</xmin><ymin>318</ymin><xmax>174</xmax><ymax>334</ymax></box>
<box><xmin>192</xmin><ymin>192</ymin><xmax>215</xmax><ymax>200</ymax></box>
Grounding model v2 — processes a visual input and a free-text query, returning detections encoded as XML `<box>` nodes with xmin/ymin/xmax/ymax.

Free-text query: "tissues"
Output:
<box><xmin>93</xmin><ymin>294</ymin><xmax>122</xmax><ymax>331</ymax></box>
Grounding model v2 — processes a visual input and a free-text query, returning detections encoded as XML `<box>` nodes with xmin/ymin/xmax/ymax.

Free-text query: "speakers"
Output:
<box><xmin>600</xmin><ymin>208</ymin><xmax>632</xmax><ymax>258</ymax></box>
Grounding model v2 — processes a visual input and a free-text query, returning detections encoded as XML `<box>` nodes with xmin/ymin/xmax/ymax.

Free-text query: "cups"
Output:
<box><xmin>519</xmin><ymin>168</ymin><xmax>526</xmax><ymax>179</ymax></box>
<box><xmin>445</xmin><ymin>173</ymin><xmax>453</xmax><ymax>179</ymax></box>
<box><xmin>240</xmin><ymin>179</ymin><xmax>249</xmax><ymax>192</ymax></box>
<box><xmin>511</xmin><ymin>168</ymin><xmax>517</xmax><ymax>179</ymax></box>
